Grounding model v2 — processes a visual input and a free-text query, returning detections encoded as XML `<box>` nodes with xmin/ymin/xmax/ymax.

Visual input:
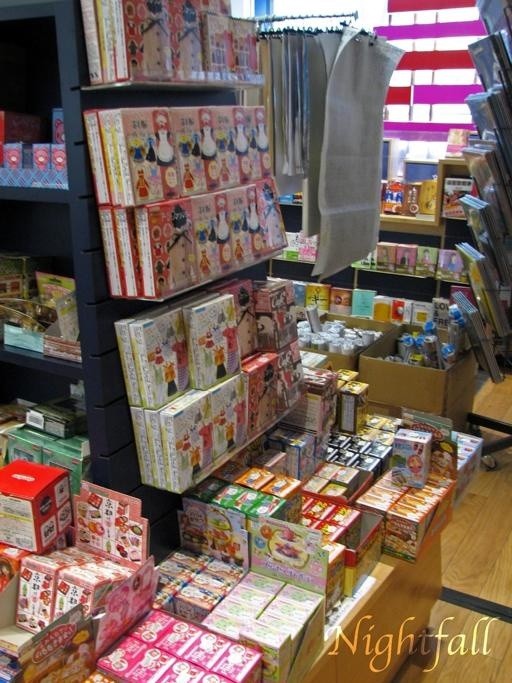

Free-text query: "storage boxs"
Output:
<box><xmin>297</xmin><ymin>311</ymin><xmax>478</xmax><ymax>438</ymax></box>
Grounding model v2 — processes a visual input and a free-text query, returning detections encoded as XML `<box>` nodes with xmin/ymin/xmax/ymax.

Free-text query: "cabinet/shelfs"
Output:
<box><xmin>1</xmin><ymin>0</ymin><xmax>171</xmax><ymax>533</ymax></box>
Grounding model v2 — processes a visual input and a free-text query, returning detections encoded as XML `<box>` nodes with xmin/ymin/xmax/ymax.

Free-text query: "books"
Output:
<box><xmin>456</xmin><ymin>0</ymin><xmax>512</xmax><ymax>384</ymax></box>
